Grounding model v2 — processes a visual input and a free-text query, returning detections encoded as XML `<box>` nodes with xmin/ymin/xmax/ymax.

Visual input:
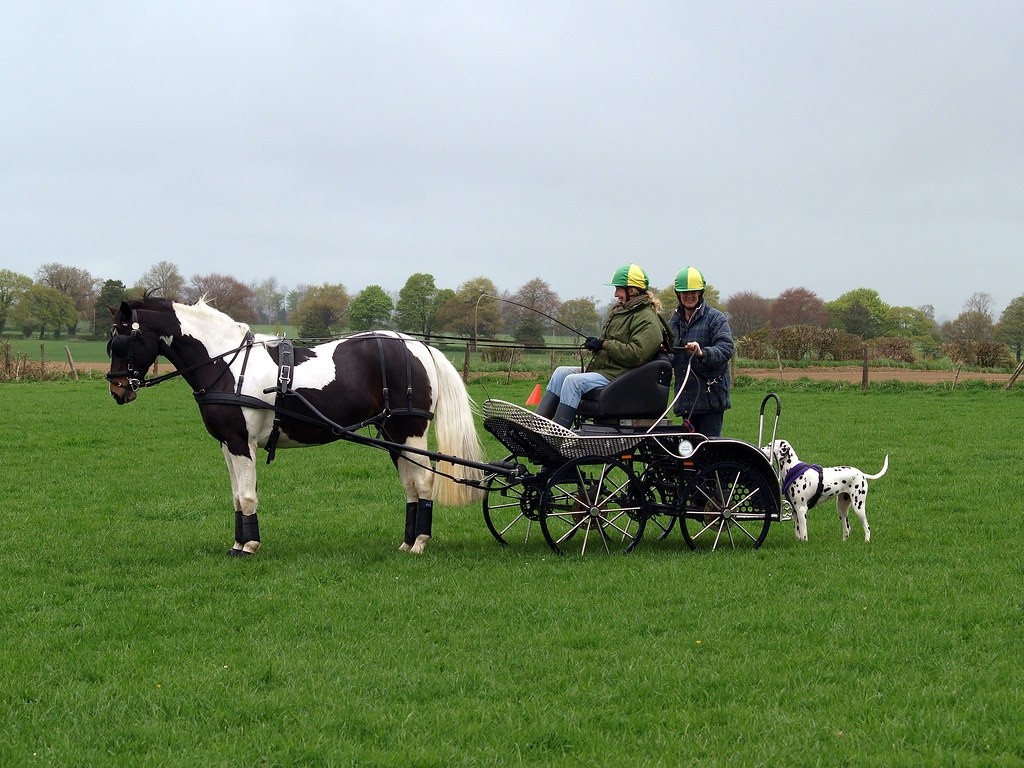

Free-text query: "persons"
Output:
<box><xmin>660</xmin><ymin>267</ymin><xmax>735</xmax><ymax>507</ymax></box>
<box><xmin>533</xmin><ymin>264</ymin><xmax>664</xmax><ymax>451</ymax></box>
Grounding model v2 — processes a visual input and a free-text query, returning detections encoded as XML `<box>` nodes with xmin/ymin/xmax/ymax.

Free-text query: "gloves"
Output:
<box><xmin>585</xmin><ymin>336</ymin><xmax>604</xmax><ymax>354</ymax></box>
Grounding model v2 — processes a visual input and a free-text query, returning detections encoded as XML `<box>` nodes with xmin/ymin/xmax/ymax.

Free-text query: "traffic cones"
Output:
<box><xmin>525</xmin><ymin>383</ymin><xmax>543</xmax><ymax>410</ymax></box>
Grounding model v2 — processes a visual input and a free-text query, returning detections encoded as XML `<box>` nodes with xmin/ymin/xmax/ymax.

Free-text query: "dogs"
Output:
<box><xmin>760</xmin><ymin>439</ymin><xmax>889</xmax><ymax>543</ymax></box>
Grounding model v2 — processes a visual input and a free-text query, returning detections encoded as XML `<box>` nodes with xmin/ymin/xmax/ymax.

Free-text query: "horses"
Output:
<box><xmin>102</xmin><ymin>284</ymin><xmax>492</xmax><ymax>561</ymax></box>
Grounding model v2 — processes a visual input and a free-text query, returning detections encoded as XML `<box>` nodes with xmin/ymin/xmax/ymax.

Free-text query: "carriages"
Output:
<box><xmin>105</xmin><ymin>297</ymin><xmax>782</xmax><ymax>555</ymax></box>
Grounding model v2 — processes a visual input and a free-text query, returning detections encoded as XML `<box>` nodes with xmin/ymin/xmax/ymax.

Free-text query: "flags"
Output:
<box><xmin>526</xmin><ymin>384</ymin><xmax>541</xmax><ymax>406</ymax></box>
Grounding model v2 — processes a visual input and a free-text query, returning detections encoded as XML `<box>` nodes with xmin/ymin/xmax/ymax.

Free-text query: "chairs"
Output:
<box><xmin>573</xmin><ymin>350</ymin><xmax>672</xmax><ymax>417</ymax></box>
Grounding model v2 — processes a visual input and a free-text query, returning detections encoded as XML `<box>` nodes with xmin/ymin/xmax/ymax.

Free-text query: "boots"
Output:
<box><xmin>513</xmin><ymin>390</ymin><xmax>577</xmax><ymax>452</ymax></box>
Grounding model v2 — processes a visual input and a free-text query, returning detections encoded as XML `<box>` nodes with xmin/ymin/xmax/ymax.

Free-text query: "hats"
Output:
<box><xmin>675</xmin><ymin>267</ymin><xmax>706</xmax><ymax>292</ymax></box>
<box><xmin>604</xmin><ymin>264</ymin><xmax>649</xmax><ymax>292</ymax></box>
